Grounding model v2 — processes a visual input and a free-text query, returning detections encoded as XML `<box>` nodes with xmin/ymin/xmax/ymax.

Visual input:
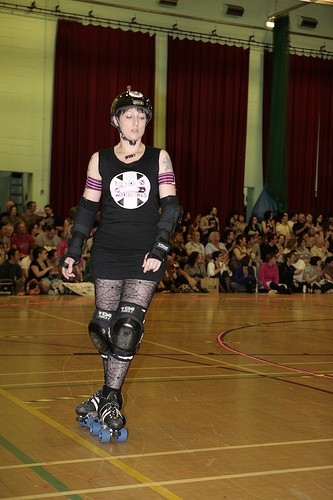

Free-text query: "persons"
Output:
<box><xmin>63</xmin><ymin>89</ymin><xmax>180</xmax><ymax>443</ymax></box>
<box><xmin>2</xmin><ymin>200</ymin><xmax>332</xmax><ymax>295</ymax></box>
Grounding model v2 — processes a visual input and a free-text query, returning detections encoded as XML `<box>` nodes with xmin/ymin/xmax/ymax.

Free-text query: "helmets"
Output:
<box><xmin>110</xmin><ymin>91</ymin><xmax>152</xmax><ymax>125</ymax></box>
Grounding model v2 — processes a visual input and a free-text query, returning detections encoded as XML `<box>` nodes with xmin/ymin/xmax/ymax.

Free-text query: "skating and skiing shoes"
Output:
<box><xmin>75</xmin><ymin>386</ymin><xmax>127</xmax><ymax>442</ymax></box>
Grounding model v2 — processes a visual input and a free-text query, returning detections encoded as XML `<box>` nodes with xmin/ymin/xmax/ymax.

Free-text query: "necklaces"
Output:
<box><xmin>125</xmin><ymin>153</ymin><xmax>135</xmax><ymax>159</ymax></box>
